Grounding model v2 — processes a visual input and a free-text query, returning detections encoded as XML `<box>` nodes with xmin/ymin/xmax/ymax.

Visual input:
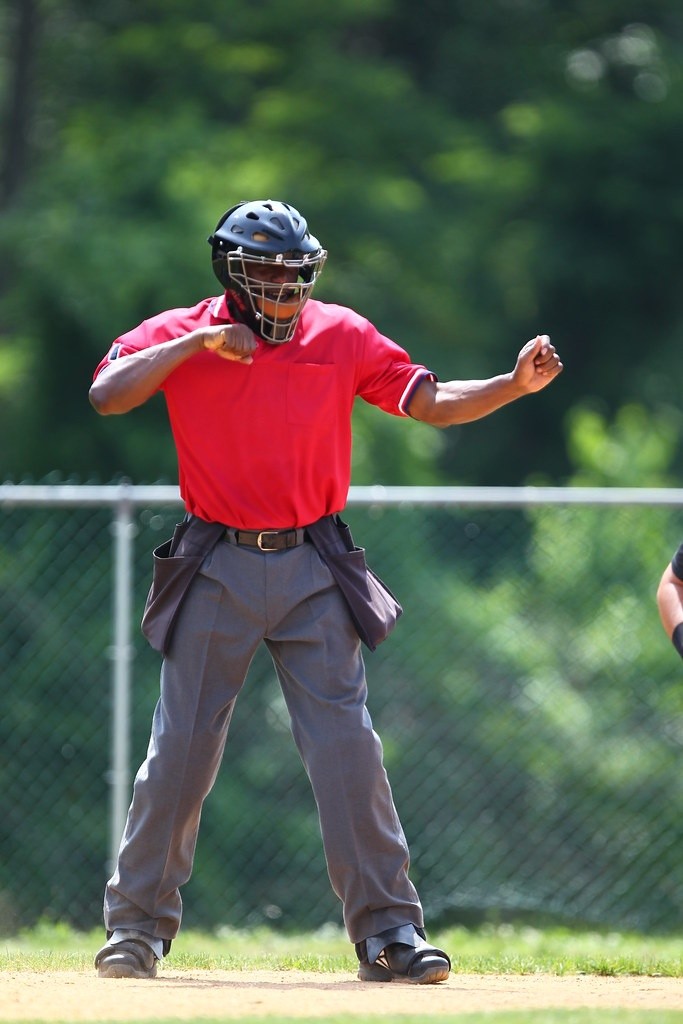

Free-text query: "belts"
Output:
<box><xmin>235</xmin><ymin>527</ymin><xmax>304</xmax><ymax>549</ymax></box>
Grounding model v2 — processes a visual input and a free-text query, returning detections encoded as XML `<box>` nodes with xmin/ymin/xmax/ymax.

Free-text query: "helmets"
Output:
<box><xmin>211</xmin><ymin>199</ymin><xmax>327</xmax><ymax>343</ymax></box>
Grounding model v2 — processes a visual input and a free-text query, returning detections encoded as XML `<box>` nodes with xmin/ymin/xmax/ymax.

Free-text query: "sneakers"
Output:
<box><xmin>355</xmin><ymin>935</ymin><xmax>452</xmax><ymax>982</ymax></box>
<box><xmin>94</xmin><ymin>929</ymin><xmax>160</xmax><ymax>977</ymax></box>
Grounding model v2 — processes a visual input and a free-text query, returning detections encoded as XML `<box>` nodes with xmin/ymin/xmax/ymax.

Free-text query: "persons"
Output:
<box><xmin>88</xmin><ymin>198</ymin><xmax>565</xmax><ymax>984</ymax></box>
<box><xmin>653</xmin><ymin>541</ymin><xmax>683</xmax><ymax>664</ymax></box>
